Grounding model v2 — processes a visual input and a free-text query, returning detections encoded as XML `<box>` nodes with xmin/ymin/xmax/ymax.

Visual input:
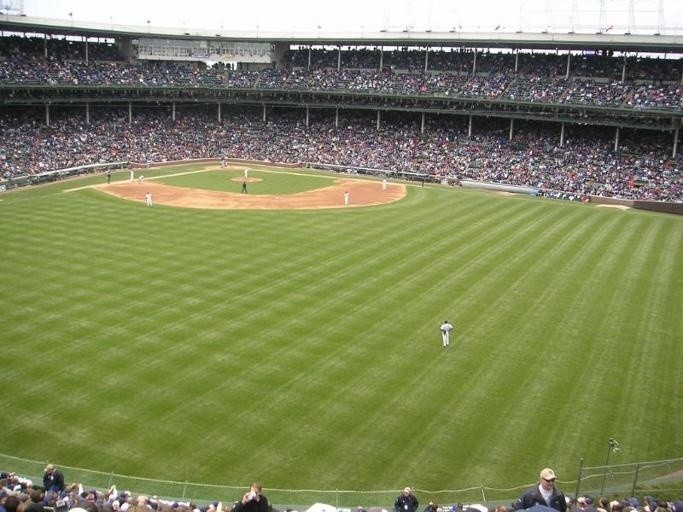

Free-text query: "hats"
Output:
<box><xmin>44</xmin><ymin>464</ymin><xmax>54</xmax><ymax>472</ymax></box>
<box><xmin>540</xmin><ymin>468</ymin><xmax>556</xmax><ymax>480</ymax></box>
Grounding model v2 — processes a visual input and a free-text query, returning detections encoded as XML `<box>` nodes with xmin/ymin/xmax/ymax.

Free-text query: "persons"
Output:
<box><xmin>439</xmin><ymin>320</ymin><xmax>453</xmax><ymax>347</ymax></box>
<box><xmin>0</xmin><ymin>463</ymin><xmax>683</xmax><ymax>512</ymax></box>
<box><xmin>383</xmin><ymin>179</ymin><xmax>386</xmax><ymax>190</ymax></box>
<box><xmin>130</xmin><ymin>170</ymin><xmax>134</xmax><ymax>181</ymax></box>
<box><xmin>343</xmin><ymin>192</ymin><xmax>349</xmax><ymax>206</ymax></box>
<box><xmin>241</xmin><ymin>181</ymin><xmax>248</xmax><ymax>194</ymax></box>
<box><xmin>145</xmin><ymin>192</ymin><xmax>152</xmax><ymax>208</ymax></box>
<box><xmin>107</xmin><ymin>171</ymin><xmax>112</xmax><ymax>184</ymax></box>
<box><xmin>244</xmin><ymin>168</ymin><xmax>248</xmax><ymax>177</ymax></box>
<box><xmin>0</xmin><ymin>34</ymin><xmax>683</xmax><ymax>203</ymax></box>
<box><xmin>139</xmin><ymin>175</ymin><xmax>144</xmax><ymax>185</ymax></box>
